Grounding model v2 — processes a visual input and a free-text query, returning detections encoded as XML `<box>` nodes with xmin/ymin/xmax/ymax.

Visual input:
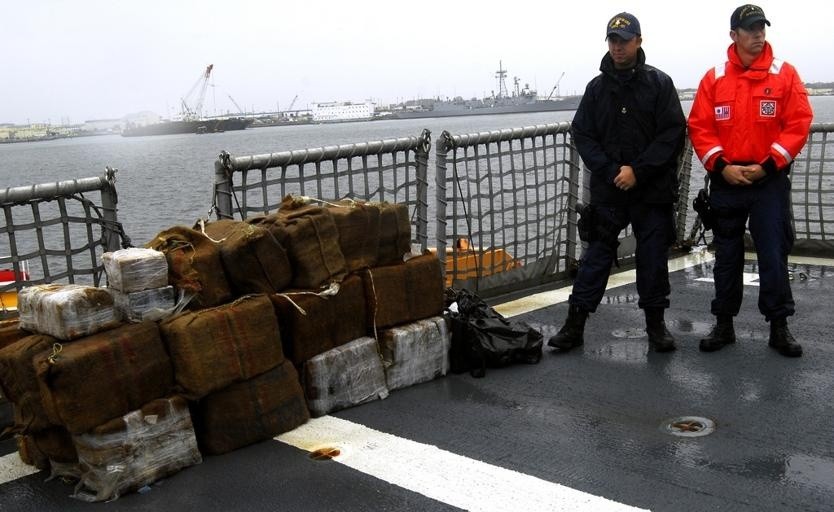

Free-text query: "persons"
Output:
<box><xmin>546</xmin><ymin>10</ymin><xmax>687</xmax><ymax>352</ymax></box>
<box><xmin>688</xmin><ymin>4</ymin><xmax>813</xmax><ymax>357</ymax></box>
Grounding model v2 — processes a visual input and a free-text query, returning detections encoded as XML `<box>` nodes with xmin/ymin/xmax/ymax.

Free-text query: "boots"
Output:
<box><xmin>548</xmin><ymin>302</ymin><xmax>590</xmax><ymax>348</ymax></box>
<box><xmin>644</xmin><ymin>307</ymin><xmax>675</xmax><ymax>351</ymax></box>
<box><xmin>767</xmin><ymin>324</ymin><xmax>801</xmax><ymax>356</ymax></box>
<box><xmin>699</xmin><ymin>315</ymin><xmax>737</xmax><ymax>353</ymax></box>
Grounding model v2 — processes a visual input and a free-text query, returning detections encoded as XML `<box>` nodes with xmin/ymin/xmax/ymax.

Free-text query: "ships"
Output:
<box><xmin>122</xmin><ymin>64</ymin><xmax>254</xmax><ymax>137</ymax></box>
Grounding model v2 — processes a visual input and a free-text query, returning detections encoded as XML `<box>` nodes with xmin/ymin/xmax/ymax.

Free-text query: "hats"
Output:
<box><xmin>606</xmin><ymin>12</ymin><xmax>641</xmax><ymax>41</ymax></box>
<box><xmin>731</xmin><ymin>4</ymin><xmax>770</xmax><ymax>27</ymax></box>
